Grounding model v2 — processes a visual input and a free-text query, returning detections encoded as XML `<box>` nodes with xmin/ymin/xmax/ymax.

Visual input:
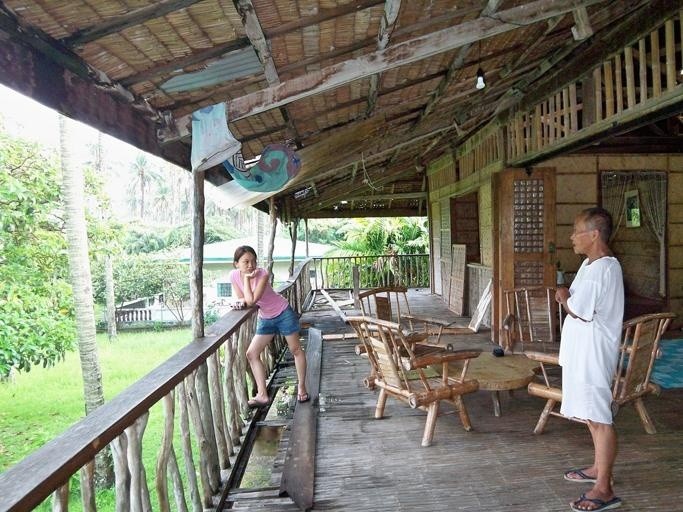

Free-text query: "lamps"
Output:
<box><xmin>475</xmin><ymin>67</ymin><xmax>486</xmax><ymax>90</ymax></box>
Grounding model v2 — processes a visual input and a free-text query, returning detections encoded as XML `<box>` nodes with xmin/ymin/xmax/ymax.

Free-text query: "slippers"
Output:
<box><xmin>295</xmin><ymin>392</ymin><xmax>310</xmax><ymax>402</ymax></box>
<box><xmin>246</xmin><ymin>397</ymin><xmax>272</xmax><ymax>407</ymax></box>
<box><xmin>563</xmin><ymin>466</ymin><xmax>614</xmax><ymax>486</ymax></box>
<box><xmin>569</xmin><ymin>491</ymin><xmax>622</xmax><ymax>512</ymax></box>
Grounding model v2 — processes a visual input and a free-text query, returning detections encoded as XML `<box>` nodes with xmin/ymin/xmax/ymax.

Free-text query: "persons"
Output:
<box><xmin>555</xmin><ymin>208</ymin><xmax>625</xmax><ymax>512</ymax></box>
<box><xmin>229</xmin><ymin>245</ymin><xmax>310</xmax><ymax>409</ymax></box>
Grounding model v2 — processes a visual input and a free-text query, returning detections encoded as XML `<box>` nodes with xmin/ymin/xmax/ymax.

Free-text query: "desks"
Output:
<box><xmin>438</xmin><ymin>351</ymin><xmax>543</xmax><ymax>417</ymax></box>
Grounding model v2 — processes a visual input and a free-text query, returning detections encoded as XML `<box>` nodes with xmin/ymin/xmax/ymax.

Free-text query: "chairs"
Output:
<box><xmin>345</xmin><ymin>316</ymin><xmax>481</xmax><ymax>448</ymax></box>
<box><xmin>501</xmin><ymin>286</ymin><xmax>567</xmax><ymax>393</ymax></box>
<box><xmin>357</xmin><ymin>287</ymin><xmax>457</xmax><ymax>389</ymax></box>
<box><xmin>524</xmin><ymin>311</ymin><xmax>677</xmax><ymax>436</ymax></box>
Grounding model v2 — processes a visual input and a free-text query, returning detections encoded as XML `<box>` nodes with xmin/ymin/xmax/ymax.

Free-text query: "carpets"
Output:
<box><xmin>617</xmin><ymin>337</ymin><xmax>683</xmax><ymax>390</ymax></box>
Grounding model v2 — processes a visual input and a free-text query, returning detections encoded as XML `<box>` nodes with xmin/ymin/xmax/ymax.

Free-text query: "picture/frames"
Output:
<box><xmin>624</xmin><ymin>189</ymin><xmax>641</xmax><ymax>228</ymax></box>
